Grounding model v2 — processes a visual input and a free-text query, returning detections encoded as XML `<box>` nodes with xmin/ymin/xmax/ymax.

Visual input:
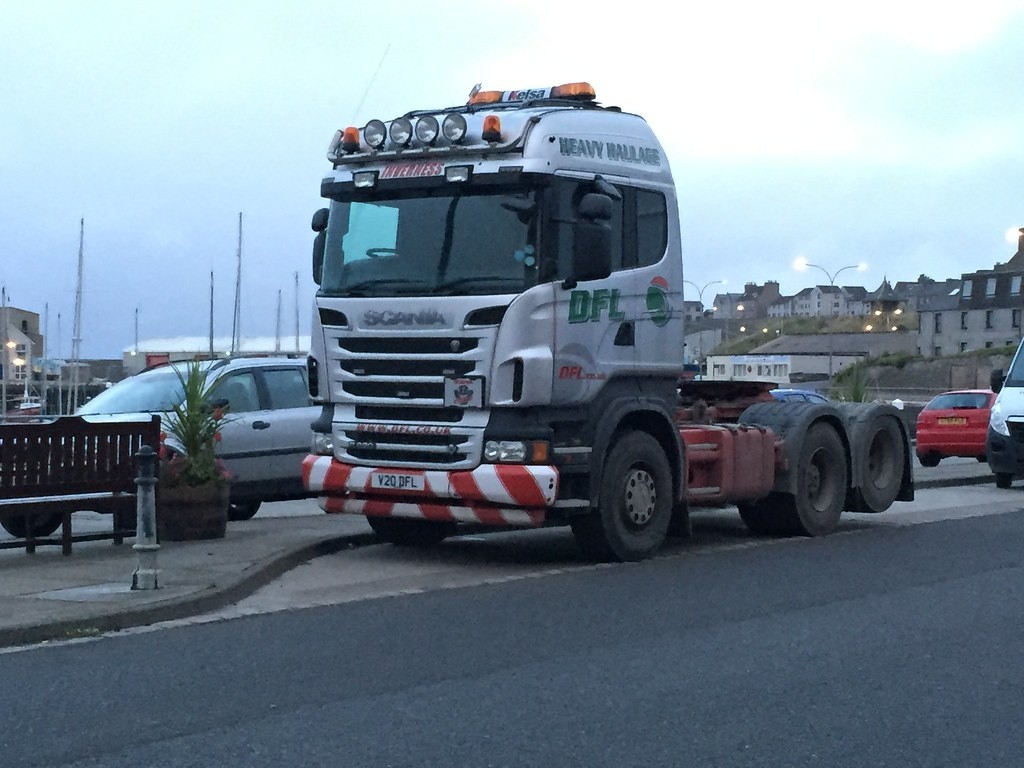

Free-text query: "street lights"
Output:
<box><xmin>797</xmin><ymin>261</ymin><xmax>866</xmax><ymax>384</ymax></box>
<box><xmin>683</xmin><ymin>280</ymin><xmax>723</xmax><ymax>380</ymax></box>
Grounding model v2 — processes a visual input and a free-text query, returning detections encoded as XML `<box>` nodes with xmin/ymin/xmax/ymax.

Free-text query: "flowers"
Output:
<box><xmin>159</xmin><ymin>351</ymin><xmax>240</xmax><ymax>483</ymax></box>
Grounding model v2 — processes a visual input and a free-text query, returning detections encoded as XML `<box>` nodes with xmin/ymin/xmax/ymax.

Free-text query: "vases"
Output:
<box><xmin>157</xmin><ymin>482</ymin><xmax>231</xmax><ymax>541</ymax></box>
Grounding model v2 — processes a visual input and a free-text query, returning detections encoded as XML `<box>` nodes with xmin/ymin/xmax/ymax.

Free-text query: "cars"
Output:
<box><xmin>916</xmin><ymin>390</ymin><xmax>998</xmax><ymax>468</ymax></box>
<box><xmin>986</xmin><ymin>337</ymin><xmax>1024</xmax><ymax>487</ymax></box>
<box><xmin>769</xmin><ymin>389</ymin><xmax>830</xmax><ymax>404</ymax></box>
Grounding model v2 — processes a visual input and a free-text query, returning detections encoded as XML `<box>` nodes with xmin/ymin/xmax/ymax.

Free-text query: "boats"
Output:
<box><xmin>5</xmin><ymin>377</ymin><xmax>43</xmax><ymax>422</ymax></box>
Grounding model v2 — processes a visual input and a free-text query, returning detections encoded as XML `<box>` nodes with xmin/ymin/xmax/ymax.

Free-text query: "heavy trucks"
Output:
<box><xmin>300</xmin><ymin>83</ymin><xmax>915</xmax><ymax>564</ymax></box>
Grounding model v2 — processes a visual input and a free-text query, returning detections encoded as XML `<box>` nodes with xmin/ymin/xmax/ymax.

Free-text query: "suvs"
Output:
<box><xmin>0</xmin><ymin>352</ymin><xmax>323</xmax><ymax>538</ymax></box>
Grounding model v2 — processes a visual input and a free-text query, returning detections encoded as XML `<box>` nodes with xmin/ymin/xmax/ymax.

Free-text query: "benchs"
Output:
<box><xmin>0</xmin><ymin>414</ymin><xmax>162</xmax><ymax>556</ymax></box>
